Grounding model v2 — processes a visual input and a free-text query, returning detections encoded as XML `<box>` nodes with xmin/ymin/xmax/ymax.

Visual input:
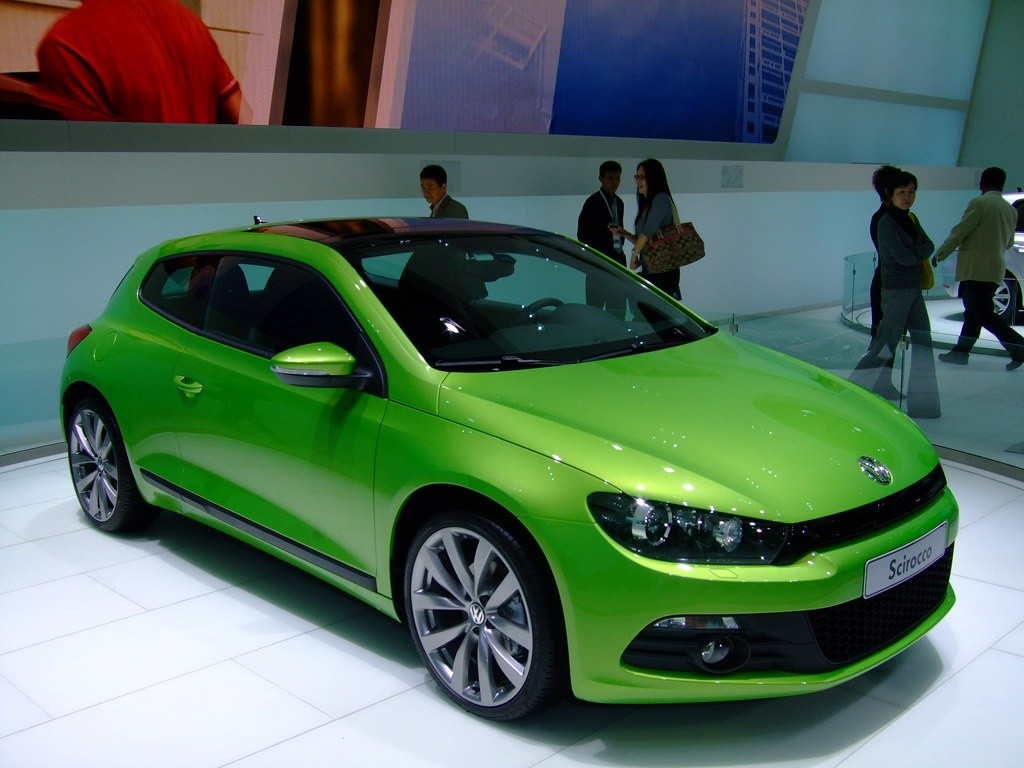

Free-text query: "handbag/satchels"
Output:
<box><xmin>636</xmin><ymin>195</ymin><xmax>705</xmax><ymax>273</ymax></box>
<box><xmin>908</xmin><ymin>212</ymin><xmax>934</xmax><ymax>289</ymax></box>
<box><xmin>448</xmin><ymin>258</ymin><xmax>489</xmax><ymax>301</ymax></box>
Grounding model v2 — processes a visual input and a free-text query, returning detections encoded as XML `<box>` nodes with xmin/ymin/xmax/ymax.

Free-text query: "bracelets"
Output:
<box><xmin>631</xmin><ymin>249</ymin><xmax>639</xmax><ymax>255</ymax></box>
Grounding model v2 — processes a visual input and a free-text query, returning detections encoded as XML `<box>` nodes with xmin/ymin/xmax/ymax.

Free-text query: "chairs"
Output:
<box><xmin>178</xmin><ymin>260</ymin><xmax>260</xmax><ymax>343</ymax></box>
<box><xmin>249</xmin><ymin>267</ymin><xmax>337</xmax><ymax>346</ymax></box>
<box><xmin>396</xmin><ymin>244</ymin><xmax>494</xmax><ymax>349</ymax></box>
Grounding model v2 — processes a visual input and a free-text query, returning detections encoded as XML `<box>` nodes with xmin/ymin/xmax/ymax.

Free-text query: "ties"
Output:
<box><xmin>612</xmin><ymin>196</ymin><xmax>623</xmax><ymax>254</ymax></box>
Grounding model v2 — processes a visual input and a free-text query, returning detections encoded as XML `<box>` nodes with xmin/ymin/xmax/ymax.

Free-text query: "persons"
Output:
<box><xmin>577</xmin><ymin>161</ymin><xmax>628</xmax><ymax>321</ymax></box>
<box><xmin>419</xmin><ymin>164</ymin><xmax>469</xmax><ymax>216</ymax></box>
<box><xmin>34</xmin><ymin>0</ymin><xmax>254</xmax><ymax>126</ymax></box>
<box><xmin>608</xmin><ymin>157</ymin><xmax>683</xmax><ymax>314</ymax></box>
<box><xmin>931</xmin><ymin>167</ymin><xmax>1024</xmax><ymax>370</ymax></box>
<box><xmin>846</xmin><ymin>164</ymin><xmax>943</xmax><ymax>419</ymax></box>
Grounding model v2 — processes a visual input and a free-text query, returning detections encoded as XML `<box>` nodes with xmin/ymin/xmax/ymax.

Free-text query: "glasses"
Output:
<box><xmin>634</xmin><ymin>175</ymin><xmax>646</xmax><ymax>182</ymax></box>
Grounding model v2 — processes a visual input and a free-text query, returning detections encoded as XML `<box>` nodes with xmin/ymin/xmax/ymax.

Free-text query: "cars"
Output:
<box><xmin>60</xmin><ymin>215</ymin><xmax>962</xmax><ymax>721</ymax></box>
<box><xmin>943</xmin><ymin>193</ymin><xmax>1024</xmax><ymax>325</ymax></box>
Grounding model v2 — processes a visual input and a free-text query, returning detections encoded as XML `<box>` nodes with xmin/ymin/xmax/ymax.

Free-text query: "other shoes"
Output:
<box><xmin>873</xmin><ymin>384</ymin><xmax>907</xmax><ymax>400</ymax></box>
<box><xmin>1006</xmin><ymin>349</ymin><xmax>1024</xmax><ymax>370</ymax></box>
<box><xmin>938</xmin><ymin>350</ymin><xmax>969</xmax><ymax>365</ymax></box>
